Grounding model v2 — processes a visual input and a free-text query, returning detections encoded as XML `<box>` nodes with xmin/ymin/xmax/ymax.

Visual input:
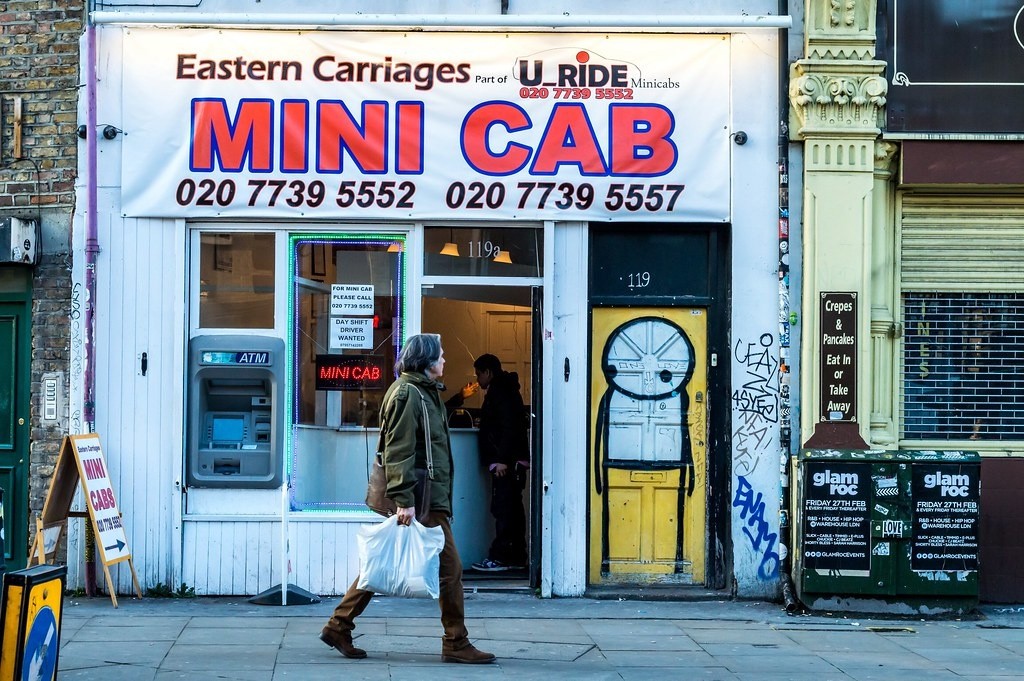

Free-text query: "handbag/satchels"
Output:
<box><xmin>356</xmin><ymin>514</ymin><xmax>445</xmax><ymax>600</ymax></box>
<box><xmin>365</xmin><ymin>452</ymin><xmax>432</xmax><ymax>523</ymax></box>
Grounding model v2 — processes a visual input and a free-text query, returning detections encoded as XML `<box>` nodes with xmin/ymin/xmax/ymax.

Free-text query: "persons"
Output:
<box><xmin>471</xmin><ymin>354</ymin><xmax>531</xmax><ymax>574</ymax></box>
<box><xmin>445</xmin><ymin>382</ymin><xmax>480</xmax><ymax>413</ymax></box>
<box><xmin>320</xmin><ymin>334</ymin><xmax>495</xmax><ymax>664</ymax></box>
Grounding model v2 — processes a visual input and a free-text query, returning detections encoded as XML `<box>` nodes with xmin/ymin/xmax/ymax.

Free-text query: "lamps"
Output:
<box><xmin>386</xmin><ymin>226</ymin><xmax>513</xmax><ymax>263</ymax></box>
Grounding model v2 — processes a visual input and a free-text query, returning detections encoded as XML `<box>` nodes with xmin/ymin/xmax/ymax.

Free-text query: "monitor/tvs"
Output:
<box><xmin>213</xmin><ymin>418</ymin><xmax>244</xmax><ymax>440</ymax></box>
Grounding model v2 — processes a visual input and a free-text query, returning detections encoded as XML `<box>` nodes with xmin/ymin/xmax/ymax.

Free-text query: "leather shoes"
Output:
<box><xmin>318</xmin><ymin>631</ymin><xmax>367</xmax><ymax>657</ymax></box>
<box><xmin>442</xmin><ymin>643</ymin><xmax>496</xmax><ymax>663</ymax></box>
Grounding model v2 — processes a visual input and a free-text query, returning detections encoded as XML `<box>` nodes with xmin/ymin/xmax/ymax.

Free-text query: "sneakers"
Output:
<box><xmin>508</xmin><ymin>561</ymin><xmax>526</xmax><ymax>570</ymax></box>
<box><xmin>471</xmin><ymin>558</ymin><xmax>508</xmax><ymax>571</ymax></box>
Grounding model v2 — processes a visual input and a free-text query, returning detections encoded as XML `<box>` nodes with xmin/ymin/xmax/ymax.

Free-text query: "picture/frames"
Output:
<box><xmin>311</xmin><ymin>242</ymin><xmax>326</xmax><ymax>275</ymax></box>
<box><xmin>310</xmin><ymin>278</ymin><xmax>327</xmax><ymax>361</ymax></box>
<box><xmin>215</xmin><ymin>234</ymin><xmax>233</xmax><ymax>271</ymax></box>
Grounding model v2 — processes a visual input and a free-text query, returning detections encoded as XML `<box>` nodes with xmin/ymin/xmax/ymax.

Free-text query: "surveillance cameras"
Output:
<box><xmin>77</xmin><ymin>125</ymin><xmax>87</xmax><ymax>139</ymax></box>
<box><xmin>735</xmin><ymin>131</ymin><xmax>748</xmax><ymax>145</ymax></box>
<box><xmin>104</xmin><ymin>126</ymin><xmax>117</xmax><ymax>139</ymax></box>
